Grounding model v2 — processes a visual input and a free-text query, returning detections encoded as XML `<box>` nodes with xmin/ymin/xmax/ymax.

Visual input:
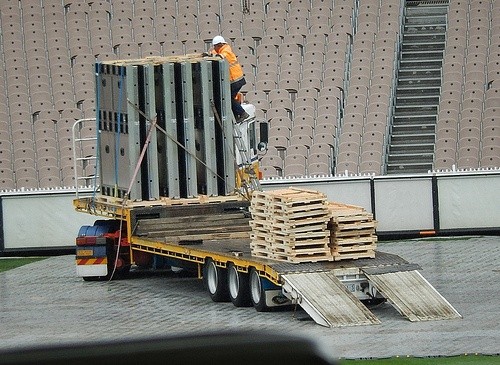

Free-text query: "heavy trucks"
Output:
<box><xmin>72</xmin><ymin>100</ymin><xmax>463</xmax><ymax>328</ymax></box>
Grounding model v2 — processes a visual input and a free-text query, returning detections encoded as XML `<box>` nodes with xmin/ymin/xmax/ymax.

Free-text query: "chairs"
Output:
<box><xmin>0</xmin><ymin>0</ymin><xmax>408</xmax><ymax>186</ymax></box>
<box><xmin>432</xmin><ymin>0</ymin><xmax>499</xmax><ymax>170</ymax></box>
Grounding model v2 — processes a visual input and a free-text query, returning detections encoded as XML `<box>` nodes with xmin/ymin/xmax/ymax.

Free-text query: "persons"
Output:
<box><xmin>202</xmin><ymin>36</ymin><xmax>249</xmax><ymax>123</ymax></box>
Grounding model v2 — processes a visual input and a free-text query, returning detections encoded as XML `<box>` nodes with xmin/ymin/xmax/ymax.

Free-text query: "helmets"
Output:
<box><xmin>213</xmin><ymin>36</ymin><xmax>227</xmax><ymax>45</ymax></box>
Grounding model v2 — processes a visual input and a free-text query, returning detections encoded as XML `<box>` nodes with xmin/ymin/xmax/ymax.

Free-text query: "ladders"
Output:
<box><xmin>231</xmin><ymin>109</ymin><xmax>263</xmax><ymax>193</ymax></box>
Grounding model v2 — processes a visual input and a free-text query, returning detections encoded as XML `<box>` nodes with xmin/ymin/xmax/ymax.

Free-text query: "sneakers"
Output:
<box><xmin>236</xmin><ymin>111</ymin><xmax>249</xmax><ymax>123</ymax></box>
